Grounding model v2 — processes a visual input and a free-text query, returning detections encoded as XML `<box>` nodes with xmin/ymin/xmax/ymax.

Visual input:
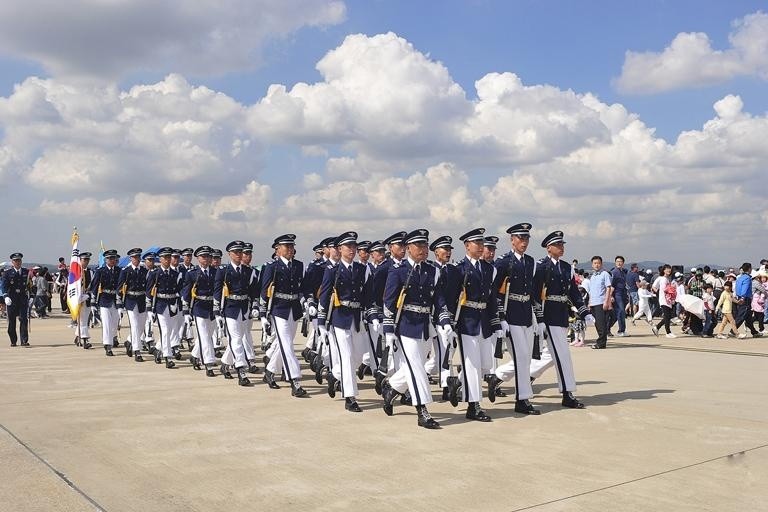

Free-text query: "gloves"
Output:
<box><xmin>585</xmin><ymin>313</ymin><xmax>596</xmax><ymax>327</ymax></box>
<box><xmin>4</xmin><ymin>296</ymin><xmax>12</xmax><ymax>307</ymax></box>
<box><xmin>78</xmin><ymin>292</ymin><xmax>548</xmax><ymax>342</ymax></box>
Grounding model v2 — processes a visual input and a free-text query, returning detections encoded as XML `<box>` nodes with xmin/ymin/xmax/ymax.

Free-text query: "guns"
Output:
<box><xmin>379</xmin><ymin>259</ymin><xmax>549</xmax><ymax>373</ymax></box>
<box><xmin>96</xmin><ymin>263</ymin><xmax>229</xmax><ymax>345</ymax></box>
<box><xmin>261</xmin><ymin>260</ymin><xmax>339</xmax><ymax>358</ymax></box>
<box><xmin>25</xmin><ymin>288</ymin><xmax>31</xmax><ymax>330</ymax></box>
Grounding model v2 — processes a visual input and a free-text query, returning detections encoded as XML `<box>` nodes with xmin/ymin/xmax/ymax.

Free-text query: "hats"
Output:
<box><xmin>541</xmin><ymin>230</ymin><xmax>567</xmax><ymax>247</ymax></box>
<box><xmin>78</xmin><ymin>251</ymin><xmax>93</xmax><ymax>259</ymax></box>
<box><xmin>646</xmin><ymin>268</ymin><xmax>653</xmax><ymax>275</ymax></box>
<box><xmin>638</xmin><ymin>280</ymin><xmax>649</xmax><ymax>287</ymax></box>
<box><xmin>101</xmin><ymin>241</ymin><xmax>253</xmax><ymax>259</ymax></box>
<box><xmin>312</xmin><ymin>228</ymin><xmax>500</xmax><ymax>253</ymax></box>
<box><xmin>506</xmin><ymin>222</ymin><xmax>532</xmax><ymax>237</ymax></box>
<box><xmin>9</xmin><ymin>251</ymin><xmax>23</xmax><ymax>262</ymax></box>
<box><xmin>270</xmin><ymin>233</ymin><xmax>297</xmax><ymax>259</ymax></box>
<box><xmin>674</xmin><ymin>264</ymin><xmax>767</xmax><ymax>279</ymax></box>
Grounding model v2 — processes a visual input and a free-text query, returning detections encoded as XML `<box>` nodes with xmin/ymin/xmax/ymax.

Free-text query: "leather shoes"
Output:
<box><xmin>10</xmin><ymin>340</ymin><xmax>17</xmax><ymax>346</ymax></box>
<box><xmin>415</xmin><ymin>404</ymin><xmax>440</xmax><ymax>428</ymax></box>
<box><xmin>497</xmin><ymin>387</ymin><xmax>506</xmax><ymax>397</ymax></box>
<box><xmin>166</xmin><ymin>345</ymin><xmax>202</xmax><ymax>370</ymax></box>
<box><xmin>261</xmin><ymin>345</ymin><xmax>306</xmax><ymax>397</ymax></box>
<box><xmin>514</xmin><ymin>400</ymin><xmax>541</xmax><ymax>416</ymax></box>
<box><xmin>374</xmin><ymin>367</ymin><xmax>412</xmax><ymax>417</ymax></box>
<box><xmin>484</xmin><ymin>373</ymin><xmax>503</xmax><ymax>402</ymax></box>
<box><xmin>562</xmin><ymin>393</ymin><xmax>584</xmax><ymax>410</ymax></box>
<box><xmin>20</xmin><ymin>341</ymin><xmax>30</xmax><ymax>346</ymax></box>
<box><xmin>466</xmin><ymin>402</ymin><xmax>491</xmax><ymax>422</ymax></box>
<box><xmin>301</xmin><ymin>348</ymin><xmax>365</xmax><ymax>412</ymax></box>
<box><xmin>74</xmin><ymin>336</ymin><xmax>164</xmax><ymax>365</ymax></box>
<box><xmin>206</xmin><ymin>350</ymin><xmax>258</xmax><ymax>385</ymax></box>
<box><xmin>428</xmin><ymin>373</ymin><xmax>462</xmax><ymax>407</ymax></box>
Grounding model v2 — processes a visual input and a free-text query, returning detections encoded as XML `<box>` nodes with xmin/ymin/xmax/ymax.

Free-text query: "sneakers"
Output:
<box><xmin>568</xmin><ymin>329</ymin><xmax>631</xmax><ymax>351</ymax></box>
<box><xmin>29</xmin><ymin>309</ymin><xmax>53</xmax><ymax>320</ymax></box>
<box><xmin>631</xmin><ymin>319</ymin><xmax>677</xmax><ymax>338</ymax></box>
<box><xmin>683</xmin><ymin>325</ymin><xmax>768</xmax><ymax>341</ymax></box>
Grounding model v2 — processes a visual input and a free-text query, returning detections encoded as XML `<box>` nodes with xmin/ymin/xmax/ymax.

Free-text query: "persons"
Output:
<box><xmin>1</xmin><ymin>223</ymin><xmax>767</xmax><ymax>429</ymax></box>
